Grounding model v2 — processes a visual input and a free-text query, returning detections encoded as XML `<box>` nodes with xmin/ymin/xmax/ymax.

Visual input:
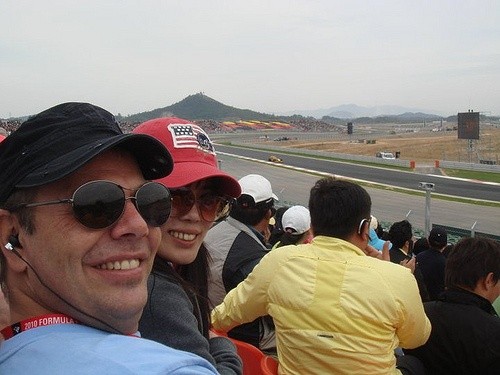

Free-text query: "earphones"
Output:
<box><xmin>5</xmin><ymin>235</ymin><xmax>21</xmax><ymax>251</ymax></box>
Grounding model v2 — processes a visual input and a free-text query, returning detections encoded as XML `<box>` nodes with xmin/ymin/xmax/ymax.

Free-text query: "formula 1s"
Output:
<box><xmin>375</xmin><ymin>151</ymin><xmax>395</xmax><ymax>160</ymax></box>
<box><xmin>274</xmin><ymin>136</ymin><xmax>289</xmax><ymax>141</ymax></box>
<box><xmin>268</xmin><ymin>156</ymin><xmax>284</xmax><ymax>163</ymax></box>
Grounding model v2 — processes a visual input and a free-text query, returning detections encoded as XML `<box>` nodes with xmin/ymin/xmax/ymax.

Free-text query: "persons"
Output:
<box><xmin>209</xmin><ymin>177</ymin><xmax>432</xmax><ymax>375</ymax></box>
<box><xmin>203</xmin><ymin>174</ymin><xmax>455</xmax><ymax>352</ymax></box>
<box><xmin>401</xmin><ymin>237</ymin><xmax>500</xmax><ymax>374</ymax></box>
<box><xmin>130</xmin><ymin>118</ymin><xmax>243</xmax><ymax>375</ymax></box>
<box><xmin>0</xmin><ymin>102</ymin><xmax>220</xmax><ymax>375</ymax></box>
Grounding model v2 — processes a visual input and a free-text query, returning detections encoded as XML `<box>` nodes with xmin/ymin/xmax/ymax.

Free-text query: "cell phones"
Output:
<box><xmin>406</xmin><ymin>240</ymin><xmax>414</xmax><ymax>262</ymax></box>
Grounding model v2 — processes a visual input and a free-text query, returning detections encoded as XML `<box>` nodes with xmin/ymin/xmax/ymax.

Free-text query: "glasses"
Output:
<box><xmin>171</xmin><ymin>186</ymin><xmax>227</xmax><ymax>223</ymax></box>
<box><xmin>261</xmin><ymin>205</ymin><xmax>277</xmax><ymax>218</ymax></box>
<box><xmin>4</xmin><ymin>180</ymin><xmax>173</xmax><ymax>229</ymax></box>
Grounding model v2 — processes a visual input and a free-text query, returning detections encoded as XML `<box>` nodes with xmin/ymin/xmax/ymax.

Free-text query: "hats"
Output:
<box><xmin>132</xmin><ymin>117</ymin><xmax>242</xmax><ymax>197</ymax></box>
<box><xmin>0</xmin><ymin>102</ymin><xmax>174</xmax><ymax>206</ymax></box>
<box><xmin>237</xmin><ymin>173</ymin><xmax>279</xmax><ymax>206</ymax></box>
<box><xmin>430</xmin><ymin>227</ymin><xmax>447</xmax><ymax>242</ymax></box>
<box><xmin>282</xmin><ymin>205</ymin><xmax>311</xmax><ymax>235</ymax></box>
